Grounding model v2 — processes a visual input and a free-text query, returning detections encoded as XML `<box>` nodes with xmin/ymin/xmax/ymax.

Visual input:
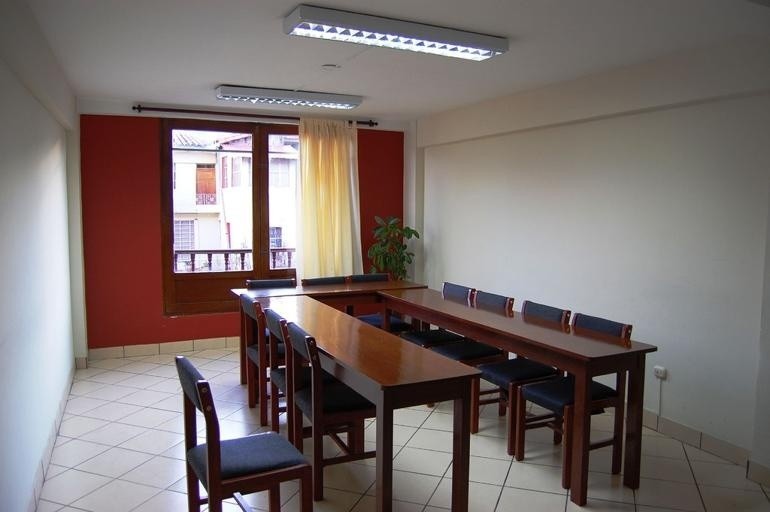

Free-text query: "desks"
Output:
<box><xmin>231</xmin><ymin>280</ymin><xmax>430</xmax><ymax>385</ymax></box>
<box><xmin>376</xmin><ymin>289</ymin><xmax>658</xmax><ymax>506</ymax></box>
<box><xmin>258</xmin><ymin>295</ymin><xmax>483</xmax><ymax>509</ymax></box>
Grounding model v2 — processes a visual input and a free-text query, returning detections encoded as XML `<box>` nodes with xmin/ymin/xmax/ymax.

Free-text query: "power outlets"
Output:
<box><xmin>652</xmin><ymin>365</ymin><xmax>670</xmax><ymax>381</ymax></box>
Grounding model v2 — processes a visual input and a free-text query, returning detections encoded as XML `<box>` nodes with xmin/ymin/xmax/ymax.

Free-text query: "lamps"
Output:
<box><xmin>282</xmin><ymin>3</ymin><xmax>511</xmax><ymax>63</ymax></box>
<box><xmin>214</xmin><ymin>83</ymin><xmax>365</xmax><ymax>110</ymax></box>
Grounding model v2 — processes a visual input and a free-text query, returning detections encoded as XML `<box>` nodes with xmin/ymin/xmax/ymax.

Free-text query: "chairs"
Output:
<box><xmin>169</xmin><ymin>353</ymin><xmax>317</xmax><ymax>510</ymax></box>
<box><xmin>229</xmin><ymin>272</ymin><xmax>661</xmax><ymax>511</ymax></box>
